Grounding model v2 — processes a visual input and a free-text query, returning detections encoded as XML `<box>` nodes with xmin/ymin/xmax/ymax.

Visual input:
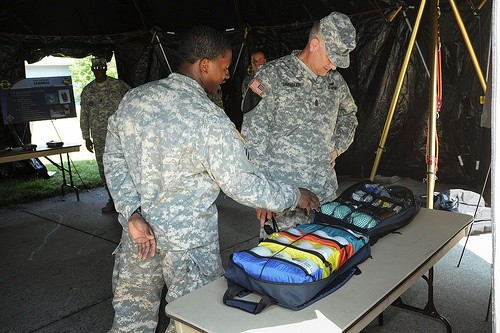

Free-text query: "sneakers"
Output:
<box><xmin>101</xmin><ymin>198</ymin><xmax>114</xmax><ymax>213</ymax></box>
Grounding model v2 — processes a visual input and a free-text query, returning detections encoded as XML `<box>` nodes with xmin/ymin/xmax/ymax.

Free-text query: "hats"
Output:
<box><xmin>91</xmin><ymin>57</ymin><xmax>108</xmax><ymax>70</ymax></box>
<box><xmin>320</xmin><ymin>12</ymin><xmax>357</xmax><ymax>68</ymax></box>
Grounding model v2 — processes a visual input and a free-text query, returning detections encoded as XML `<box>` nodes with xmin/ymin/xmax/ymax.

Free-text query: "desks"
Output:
<box><xmin>165</xmin><ymin>208</ymin><xmax>473</xmax><ymax>333</ymax></box>
<box><xmin>0</xmin><ymin>144</ymin><xmax>81</xmax><ymax>202</ymax></box>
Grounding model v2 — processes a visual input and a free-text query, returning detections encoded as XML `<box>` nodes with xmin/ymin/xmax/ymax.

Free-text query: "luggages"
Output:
<box><xmin>223</xmin><ymin>179</ymin><xmax>421</xmax><ymax>315</ymax></box>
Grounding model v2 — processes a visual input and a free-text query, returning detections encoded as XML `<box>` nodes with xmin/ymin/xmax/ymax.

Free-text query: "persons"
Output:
<box><xmin>240</xmin><ymin>11</ymin><xmax>358</xmax><ymax>242</ymax></box>
<box><xmin>80</xmin><ymin>24</ymin><xmax>321</xmax><ymax>333</ymax></box>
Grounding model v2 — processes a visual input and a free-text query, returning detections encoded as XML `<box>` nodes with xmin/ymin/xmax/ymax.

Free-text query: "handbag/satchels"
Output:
<box><xmin>419</xmin><ymin>188</ymin><xmax>485</xmax><ymax>217</ymax></box>
